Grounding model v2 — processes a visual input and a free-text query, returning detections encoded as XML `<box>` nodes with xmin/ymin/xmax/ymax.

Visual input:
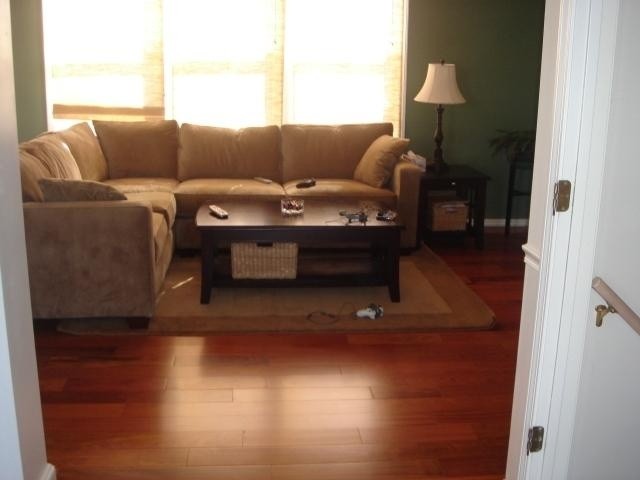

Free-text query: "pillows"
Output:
<box><xmin>40</xmin><ymin>171</ymin><xmax>128</xmax><ymax>208</ymax></box>
<box><xmin>351</xmin><ymin>132</ymin><xmax>411</xmax><ymax>189</ymax></box>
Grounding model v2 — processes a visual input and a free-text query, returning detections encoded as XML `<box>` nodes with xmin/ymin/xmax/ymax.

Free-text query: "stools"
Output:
<box><xmin>503</xmin><ymin>152</ymin><xmax>536</xmax><ymax>237</ymax></box>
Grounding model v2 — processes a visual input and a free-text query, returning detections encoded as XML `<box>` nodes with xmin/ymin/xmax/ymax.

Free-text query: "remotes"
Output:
<box><xmin>209</xmin><ymin>203</ymin><xmax>229</xmax><ymax>219</ymax></box>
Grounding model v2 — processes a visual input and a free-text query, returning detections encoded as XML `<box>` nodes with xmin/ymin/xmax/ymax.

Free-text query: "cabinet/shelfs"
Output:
<box><xmin>416</xmin><ymin>162</ymin><xmax>489</xmax><ymax>252</ymax></box>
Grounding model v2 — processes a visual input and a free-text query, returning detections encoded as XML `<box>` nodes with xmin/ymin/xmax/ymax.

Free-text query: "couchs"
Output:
<box><xmin>17</xmin><ymin>119</ymin><xmax>425</xmax><ymax>329</ymax></box>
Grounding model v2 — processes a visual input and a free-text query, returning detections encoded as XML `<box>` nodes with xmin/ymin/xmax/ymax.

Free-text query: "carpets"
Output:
<box><xmin>147</xmin><ymin>240</ymin><xmax>498</xmax><ymax>332</ymax></box>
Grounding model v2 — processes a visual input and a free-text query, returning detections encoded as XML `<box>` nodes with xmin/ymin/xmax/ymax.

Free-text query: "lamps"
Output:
<box><xmin>412</xmin><ymin>57</ymin><xmax>468</xmax><ymax>173</ymax></box>
